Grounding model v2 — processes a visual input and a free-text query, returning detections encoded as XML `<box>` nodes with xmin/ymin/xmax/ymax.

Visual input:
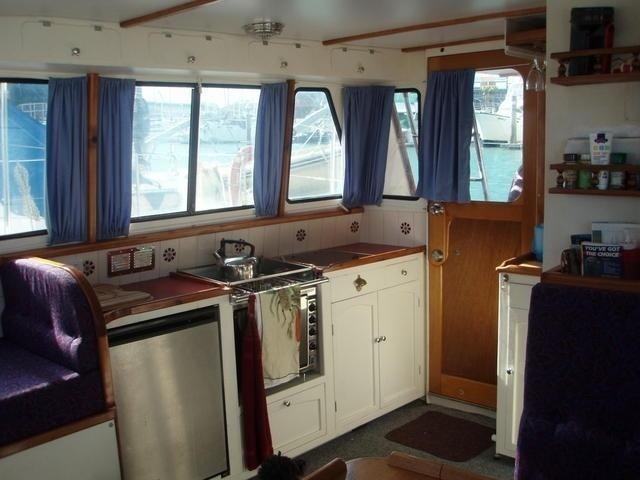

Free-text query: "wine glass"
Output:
<box><xmin>523</xmin><ymin>54</ymin><xmax>547</xmax><ymax>91</ymax></box>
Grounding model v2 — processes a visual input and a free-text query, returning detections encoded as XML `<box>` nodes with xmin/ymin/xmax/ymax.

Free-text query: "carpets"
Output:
<box><xmin>384</xmin><ymin>411</ymin><xmax>495</xmax><ymax>462</ymax></box>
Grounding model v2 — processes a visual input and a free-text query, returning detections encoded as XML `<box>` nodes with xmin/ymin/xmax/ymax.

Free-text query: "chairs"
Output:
<box><xmin>1</xmin><ymin>255</ymin><xmax>112</xmax><ymax>449</ymax></box>
<box><xmin>509</xmin><ymin>283</ymin><xmax>639</xmax><ymax>479</ymax></box>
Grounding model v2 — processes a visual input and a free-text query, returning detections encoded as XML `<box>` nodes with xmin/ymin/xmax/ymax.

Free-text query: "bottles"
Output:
<box><xmin>561</xmin><ymin>233</ymin><xmax>591</xmax><ymax>276</ymax></box>
<box><xmin>560</xmin><ymin>151</ymin><xmax>626</xmax><ymax>190</ymax></box>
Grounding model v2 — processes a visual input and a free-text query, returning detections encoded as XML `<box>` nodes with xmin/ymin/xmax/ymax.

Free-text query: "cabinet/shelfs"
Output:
<box><xmin>318</xmin><ymin>252</ymin><xmax>426</xmax><ymax>444</ymax></box>
<box><xmin>546</xmin><ymin>44</ymin><xmax>640</xmax><ymax>198</ymax></box>
<box><xmin>264</xmin><ymin>380</ymin><xmax>334</xmax><ymax>461</ymax></box>
<box><xmin>490</xmin><ymin>275</ymin><xmax>541</xmax><ymax>462</ymax></box>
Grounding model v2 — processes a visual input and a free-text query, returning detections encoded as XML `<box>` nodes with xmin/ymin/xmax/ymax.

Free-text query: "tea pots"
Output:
<box><xmin>214</xmin><ymin>238</ymin><xmax>260</xmax><ymax>282</ymax></box>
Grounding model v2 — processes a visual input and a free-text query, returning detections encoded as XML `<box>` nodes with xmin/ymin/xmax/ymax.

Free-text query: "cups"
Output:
<box><xmin>535</xmin><ymin>224</ymin><xmax>544</xmax><ymax>259</ymax></box>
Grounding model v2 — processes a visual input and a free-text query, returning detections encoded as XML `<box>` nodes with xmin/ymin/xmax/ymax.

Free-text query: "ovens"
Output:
<box><xmin>232</xmin><ymin>279</ymin><xmax>328</xmax><ymax>394</ymax></box>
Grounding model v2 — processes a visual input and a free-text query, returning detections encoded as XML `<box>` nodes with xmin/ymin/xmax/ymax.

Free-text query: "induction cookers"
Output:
<box><xmin>176</xmin><ymin>255</ymin><xmax>314</xmax><ymax>287</ymax></box>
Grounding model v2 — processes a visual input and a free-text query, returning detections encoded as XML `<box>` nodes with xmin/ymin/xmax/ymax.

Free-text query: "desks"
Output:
<box><xmin>296</xmin><ymin>456</ymin><xmax>495</xmax><ymax>480</ymax></box>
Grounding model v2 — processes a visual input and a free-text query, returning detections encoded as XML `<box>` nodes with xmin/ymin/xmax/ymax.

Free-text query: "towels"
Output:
<box><xmin>257</xmin><ymin>284</ymin><xmax>302</xmax><ymax>389</ymax></box>
